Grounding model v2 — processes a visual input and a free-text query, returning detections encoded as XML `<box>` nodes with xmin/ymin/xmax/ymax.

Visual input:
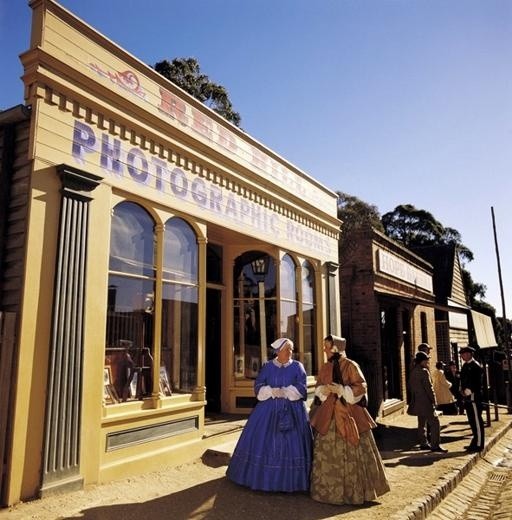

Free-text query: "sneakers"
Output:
<box><xmin>420</xmin><ymin>441</ymin><xmax>485</xmax><ymax>452</ymax></box>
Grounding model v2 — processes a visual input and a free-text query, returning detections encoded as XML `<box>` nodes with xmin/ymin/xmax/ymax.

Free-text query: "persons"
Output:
<box><xmin>444</xmin><ymin>360</ymin><xmax>465</xmax><ymax>415</ymax></box>
<box><xmin>308</xmin><ymin>333</ymin><xmax>391</xmax><ymax>506</ymax></box>
<box><xmin>225</xmin><ymin>337</ymin><xmax>312</xmax><ymax>495</ymax></box>
<box><xmin>142</xmin><ymin>346</ymin><xmax>154</xmax><ymax>396</ymax></box>
<box><xmin>406</xmin><ymin>350</ymin><xmax>449</xmax><ymax>453</ymax></box>
<box><xmin>411</xmin><ymin>343</ymin><xmax>433</xmax><ymax>443</ymax></box>
<box><xmin>458</xmin><ymin>346</ymin><xmax>486</xmax><ymax>454</ymax></box>
<box><xmin>108</xmin><ymin>339</ymin><xmax>136</xmax><ymax>403</ymax></box>
<box><xmin>430</xmin><ymin>359</ymin><xmax>458</xmax><ymax>416</ymax></box>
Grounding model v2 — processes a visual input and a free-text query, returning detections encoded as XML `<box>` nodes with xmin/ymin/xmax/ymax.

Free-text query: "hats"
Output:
<box><xmin>412</xmin><ymin>343</ymin><xmax>476</xmax><ymax>363</ymax></box>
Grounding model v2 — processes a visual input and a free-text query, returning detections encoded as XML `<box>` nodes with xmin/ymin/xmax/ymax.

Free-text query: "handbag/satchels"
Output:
<box><xmin>276</xmin><ymin>407</ymin><xmax>296</xmax><ymax>432</ymax></box>
<box><xmin>341</xmin><ymin>416</ymin><xmax>360</xmax><ymax>447</ymax></box>
